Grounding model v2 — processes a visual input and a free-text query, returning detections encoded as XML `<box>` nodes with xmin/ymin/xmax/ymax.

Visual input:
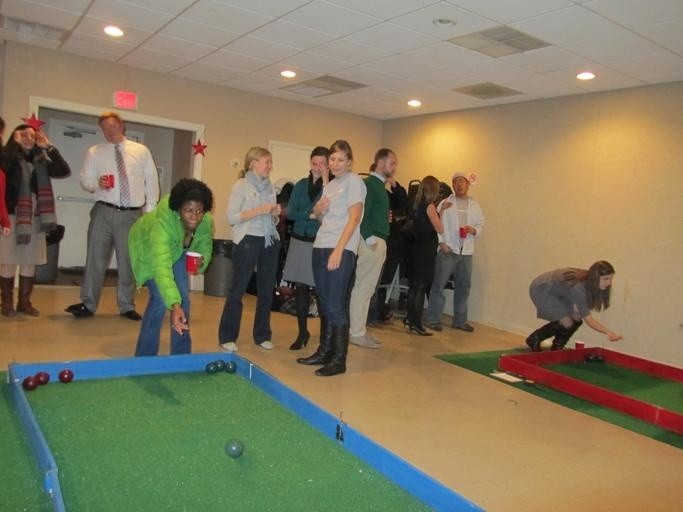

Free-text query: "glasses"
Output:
<box><xmin>184</xmin><ymin>208</ymin><xmax>203</xmax><ymax>215</ymax></box>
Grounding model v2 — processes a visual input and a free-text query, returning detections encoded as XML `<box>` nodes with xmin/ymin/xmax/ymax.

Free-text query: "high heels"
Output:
<box><xmin>290</xmin><ymin>331</ymin><xmax>310</xmax><ymax>350</ymax></box>
<box><xmin>403</xmin><ymin>318</ymin><xmax>426</xmax><ymax>331</ymax></box>
<box><xmin>409</xmin><ymin>325</ymin><xmax>432</xmax><ymax>336</ymax></box>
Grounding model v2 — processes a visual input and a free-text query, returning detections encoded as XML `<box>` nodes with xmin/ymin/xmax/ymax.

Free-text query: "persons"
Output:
<box><xmin>0</xmin><ymin>118</ymin><xmax>12</xmax><ymax>237</ymax></box>
<box><xmin>368</xmin><ymin>172</ymin><xmax>484</xmax><ymax>337</ymax></box>
<box><xmin>0</xmin><ymin>123</ymin><xmax>71</xmax><ymax>317</ymax></box>
<box><xmin>525</xmin><ymin>259</ymin><xmax>623</xmax><ymax>352</ymax></box>
<box><xmin>126</xmin><ymin>178</ymin><xmax>215</xmax><ymax>356</ymax></box>
<box><xmin>216</xmin><ymin>139</ymin><xmax>397</xmax><ymax>376</ymax></box>
<box><xmin>62</xmin><ymin>111</ymin><xmax>161</xmax><ymax>321</ymax></box>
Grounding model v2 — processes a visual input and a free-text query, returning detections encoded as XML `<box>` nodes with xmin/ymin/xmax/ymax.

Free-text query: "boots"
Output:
<box><xmin>17</xmin><ymin>276</ymin><xmax>39</xmax><ymax>316</ymax></box>
<box><xmin>551</xmin><ymin>319</ymin><xmax>583</xmax><ymax>351</ymax></box>
<box><xmin>297</xmin><ymin>320</ymin><xmax>332</xmax><ymax>365</ymax></box>
<box><xmin>0</xmin><ymin>276</ymin><xmax>16</xmax><ymax>317</ymax></box>
<box><xmin>316</xmin><ymin>326</ymin><xmax>348</xmax><ymax>376</ymax></box>
<box><xmin>526</xmin><ymin>320</ymin><xmax>567</xmax><ymax>352</ymax></box>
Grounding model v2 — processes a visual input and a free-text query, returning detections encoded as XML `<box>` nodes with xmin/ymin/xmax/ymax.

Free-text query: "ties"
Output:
<box><xmin>115</xmin><ymin>146</ymin><xmax>131</xmax><ymax>208</ymax></box>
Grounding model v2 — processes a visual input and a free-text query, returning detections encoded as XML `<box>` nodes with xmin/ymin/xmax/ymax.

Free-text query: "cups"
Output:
<box><xmin>185</xmin><ymin>252</ymin><xmax>201</xmax><ymax>274</ymax></box>
<box><xmin>574</xmin><ymin>341</ymin><xmax>584</xmax><ymax>349</ymax></box>
<box><xmin>459</xmin><ymin>227</ymin><xmax>467</xmax><ymax>238</ymax></box>
<box><xmin>103</xmin><ymin>174</ymin><xmax>114</xmax><ymax>188</ymax></box>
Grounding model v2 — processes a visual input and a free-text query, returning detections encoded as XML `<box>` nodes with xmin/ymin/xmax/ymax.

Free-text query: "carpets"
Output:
<box><xmin>1</xmin><ymin>365</ymin><xmax>438</xmax><ymax>511</ymax></box>
<box><xmin>432</xmin><ymin>345</ymin><xmax>682</xmax><ymax>450</ymax></box>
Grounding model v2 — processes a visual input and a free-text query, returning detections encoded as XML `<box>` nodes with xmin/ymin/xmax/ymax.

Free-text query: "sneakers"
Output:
<box><xmin>348</xmin><ymin>332</ymin><xmax>381</xmax><ymax>350</ymax></box>
<box><xmin>424</xmin><ymin>322</ymin><xmax>442</xmax><ymax>330</ymax></box>
<box><xmin>368</xmin><ymin>320</ymin><xmax>377</xmax><ymax>327</ymax></box>
<box><xmin>452</xmin><ymin>323</ymin><xmax>473</xmax><ymax>332</ymax></box>
<box><xmin>260</xmin><ymin>340</ymin><xmax>274</xmax><ymax>349</ymax></box>
<box><xmin>220</xmin><ymin>342</ymin><xmax>238</xmax><ymax>352</ymax></box>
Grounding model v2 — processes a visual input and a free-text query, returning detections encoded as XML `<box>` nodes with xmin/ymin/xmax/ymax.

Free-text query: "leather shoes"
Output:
<box><xmin>120</xmin><ymin>311</ymin><xmax>141</xmax><ymax>320</ymax></box>
<box><xmin>68</xmin><ymin>303</ymin><xmax>93</xmax><ymax>316</ymax></box>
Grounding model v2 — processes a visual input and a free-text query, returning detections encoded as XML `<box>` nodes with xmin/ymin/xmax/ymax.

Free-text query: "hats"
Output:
<box><xmin>452</xmin><ymin>174</ymin><xmax>468</xmax><ymax>183</ymax></box>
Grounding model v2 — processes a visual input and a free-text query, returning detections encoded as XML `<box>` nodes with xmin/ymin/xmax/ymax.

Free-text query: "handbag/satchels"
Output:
<box><xmin>399</xmin><ymin>215</ymin><xmax>418</xmax><ymax>243</ymax></box>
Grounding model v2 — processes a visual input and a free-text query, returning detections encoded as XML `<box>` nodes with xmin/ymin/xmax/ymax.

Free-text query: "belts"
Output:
<box><xmin>96</xmin><ymin>201</ymin><xmax>141</xmax><ymax>211</ymax></box>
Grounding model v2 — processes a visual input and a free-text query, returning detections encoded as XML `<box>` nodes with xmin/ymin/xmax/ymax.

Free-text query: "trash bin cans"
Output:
<box><xmin>35</xmin><ymin>225</ymin><xmax>63</xmax><ymax>283</ymax></box>
<box><xmin>205</xmin><ymin>239</ymin><xmax>236</xmax><ymax>297</ymax></box>
